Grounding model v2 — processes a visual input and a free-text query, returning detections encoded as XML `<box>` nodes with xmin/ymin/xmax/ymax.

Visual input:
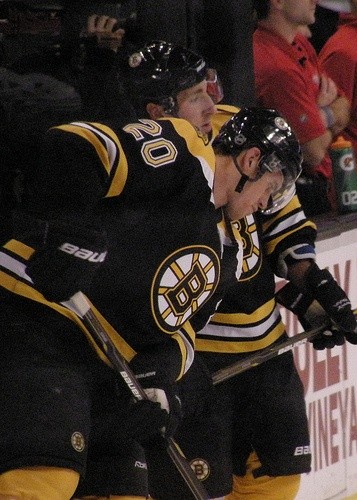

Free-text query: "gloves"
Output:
<box><xmin>128</xmin><ymin>333</ymin><xmax>188</xmax><ymax>389</ymax></box>
<box><xmin>25</xmin><ymin>212</ymin><xmax>108</xmax><ymax>303</ymax></box>
<box><xmin>276</xmin><ymin>267</ymin><xmax>357</xmax><ymax>349</ymax></box>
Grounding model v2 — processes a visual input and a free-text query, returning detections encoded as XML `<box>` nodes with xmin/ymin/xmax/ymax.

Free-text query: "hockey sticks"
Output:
<box><xmin>61</xmin><ymin>291</ymin><xmax>207</xmax><ymax>500</ymax></box>
<box><xmin>206</xmin><ymin>308</ymin><xmax>356</xmax><ymax>385</ymax></box>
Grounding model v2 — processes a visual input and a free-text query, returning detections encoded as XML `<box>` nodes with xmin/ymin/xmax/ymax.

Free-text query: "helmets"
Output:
<box><xmin>212</xmin><ymin>104</ymin><xmax>303</xmax><ymax>182</ymax></box>
<box><xmin>120</xmin><ymin>38</ymin><xmax>207</xmax><ymax>101</ymax></box>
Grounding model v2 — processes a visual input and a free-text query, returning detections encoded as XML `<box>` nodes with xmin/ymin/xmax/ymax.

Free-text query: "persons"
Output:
<box><xmin>0</xmin><ymin>104</ymin><xmax>306</xmax><ymax>499</ymax></box>
<box><xmin>124</xmin><ymin>35</ymin><xmax>357</xmax><ymax>499</ymax></box>
<box><xmin>0</xmin><ymin>0</ymin><xmax>357</xmax><ymax>224</ymax></box>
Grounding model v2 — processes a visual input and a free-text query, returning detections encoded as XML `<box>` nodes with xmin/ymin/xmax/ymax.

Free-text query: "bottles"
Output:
<box><xmin>330</xmin><ymin>136</ymin><xmax>357</xmax><ymax>213</ymax></box>
